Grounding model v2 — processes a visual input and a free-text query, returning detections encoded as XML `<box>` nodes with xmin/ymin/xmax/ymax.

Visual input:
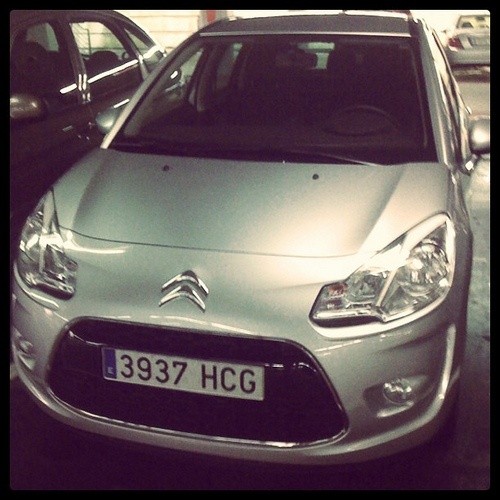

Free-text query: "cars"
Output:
<box><xmin>9</xmin><ymin>10</ymin><xmax>187</xmax><ymax>250</ymax></box>
<box><xmin>441</xmin><ymin>12</ymin><xmax>492</xmax><ymax>69</ymax></box>
<box><xmin>9</xmin><ymin>10</ymin><xmax>491</xmax><ymax>469</ymax></box>
